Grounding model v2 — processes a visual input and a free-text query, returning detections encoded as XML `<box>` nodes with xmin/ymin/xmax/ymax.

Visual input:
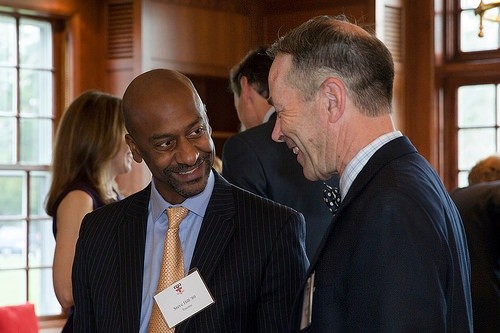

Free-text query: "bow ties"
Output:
<box><xmin>322</xmin><ymin>182</ymin><xmax>341</xmax><ymax>216</ymax></box>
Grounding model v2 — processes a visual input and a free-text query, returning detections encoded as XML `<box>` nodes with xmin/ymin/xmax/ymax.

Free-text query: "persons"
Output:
<box><xmin>70</xmin><ymin>68</ymin><xmax>310</xmax><ymax>333</ymax></box>
<box><xmin>223</xmin><ymin>16</ymin><xmax>500</xmax><ymax>333</ymax></box>
<box><xmin>46</xmin><ymin>90</ymin><xmax>131</xmax><ymax>333</ymax></box>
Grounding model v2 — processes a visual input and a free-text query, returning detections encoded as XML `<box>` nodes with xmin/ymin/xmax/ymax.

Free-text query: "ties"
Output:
<box><xmin>146</xmin><ymin>207</ymin><xmax>189</xmax><ymax>333</ymax></box>
